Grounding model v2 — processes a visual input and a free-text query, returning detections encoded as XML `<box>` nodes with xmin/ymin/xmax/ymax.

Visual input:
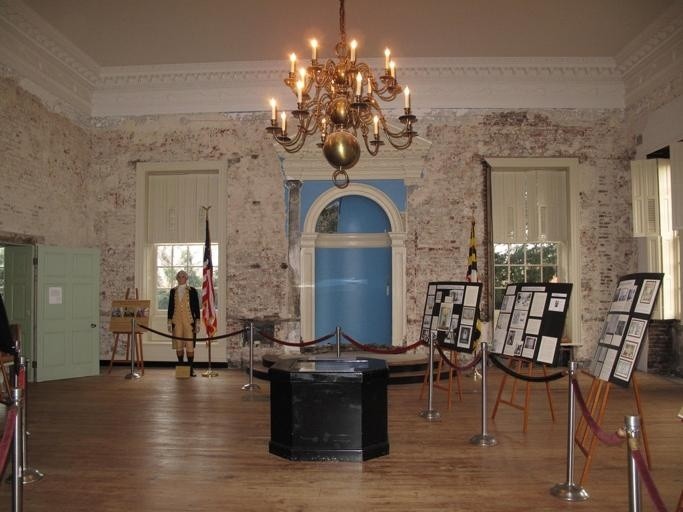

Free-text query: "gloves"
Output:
<box><xmin>168</xmin><ymin>324</ymin><xmax>172</xmax><ymax>332</ymax></box>
<box><xmin>195</xmin><ymin>320</ymin><xmax>200</xmax><ymax>331</ymax></box>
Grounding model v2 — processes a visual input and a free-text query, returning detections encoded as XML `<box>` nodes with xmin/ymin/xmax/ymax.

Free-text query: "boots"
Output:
<box><xmin>187</xmin><ymin>356</ymin><xmax>196</xmax><ymax>377</ymax></box>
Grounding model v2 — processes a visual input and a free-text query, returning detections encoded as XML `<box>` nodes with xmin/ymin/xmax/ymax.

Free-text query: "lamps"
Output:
<box><xmin>265</xmin><ymin>0</ymin><xmax>418</xmax><ymax>189</ymax></box>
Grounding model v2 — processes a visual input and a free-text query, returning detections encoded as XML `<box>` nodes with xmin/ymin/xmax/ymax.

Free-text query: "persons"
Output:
<box><xmin>168</xmin><ymin>270</ymin><xmax>201</xmax><ymax>377</ymax></box>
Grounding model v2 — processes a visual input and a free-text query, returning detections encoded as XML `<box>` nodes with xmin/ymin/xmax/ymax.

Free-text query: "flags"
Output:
<box><xmin>201</xmin><ymin>220</ymin><xmax>218</xmax><ymax>347</ymax></box>
<box><xmin>465</xmin><ymin>221</ymin><xmax>483</xmax><ymax>351</ymax></box>
